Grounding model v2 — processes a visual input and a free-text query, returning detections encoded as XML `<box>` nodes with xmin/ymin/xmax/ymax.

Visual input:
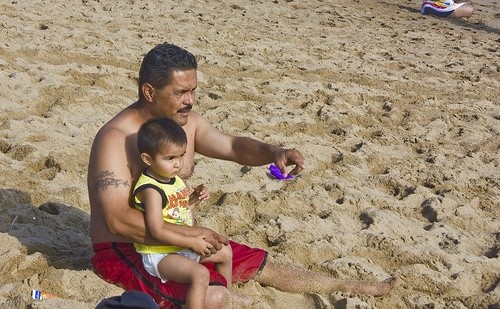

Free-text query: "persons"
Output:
<box><xmin>131</xmin><ymin>117</ymin><xmax>254</xmax><ymax>309</ymax></box>
<box><xmin>88</xmin><ymin>43</ymin><xmax>396</xmax><ymax>309</ymax></box>
<box><xmin>421</xmin><ymin>0</ymin><xmax>474</xmax><ymax>18</ymax></box>
